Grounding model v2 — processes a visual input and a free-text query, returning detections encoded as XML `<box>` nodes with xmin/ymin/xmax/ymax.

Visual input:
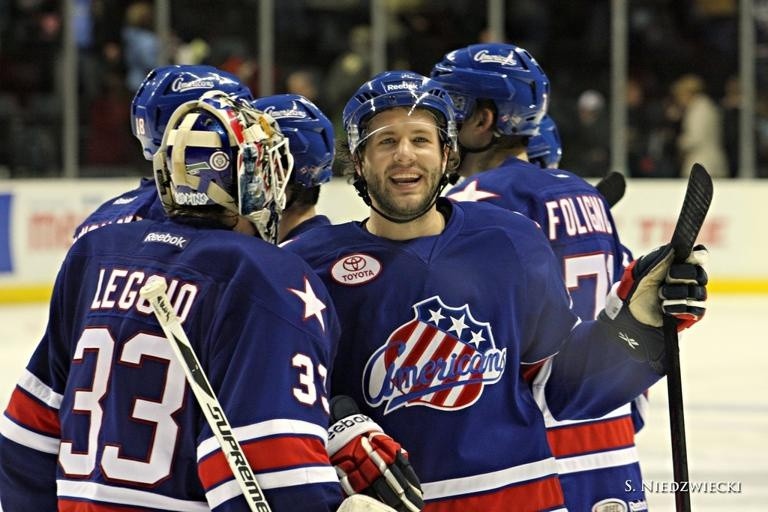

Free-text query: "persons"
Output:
<box><xmin>1</xmin><ymin>1</ymin><xmax>766</xmax><ymax>512</ymax></box>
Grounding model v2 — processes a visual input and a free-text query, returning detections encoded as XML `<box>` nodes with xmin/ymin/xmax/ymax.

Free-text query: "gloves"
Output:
<box><xmin>597</xmin><ymin>242</ymin><xmax>708</xmax><ymax>377</ymax></box>
<box><xmin>327</xmin><ymin>395</ymin><xmax>424</xmax><ymax>512</ymax></box>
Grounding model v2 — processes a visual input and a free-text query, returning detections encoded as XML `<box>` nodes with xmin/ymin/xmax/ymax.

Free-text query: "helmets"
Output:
<box><xmin>430</xmin><ymin>44</ymin><xmax>563</xmax><ymax>169</ymax></box>
<box><xmin>130</xmin><ymin>65</ymin><xmax>254</xmax><ymax>162</ymax></box>
<box><xmin>346</xmin><ymin>71</ymin><xmax>458</xmax><ymax>158</ymax></box>
<box><xmin>251</xmin><ymin>94</ymin><xmax>335</xmax><ymax>185</ymax></box>
<box><xmin>151</xmin><ymin>97</ymin><xmax>293</xmax><ymax>244</ymax></box>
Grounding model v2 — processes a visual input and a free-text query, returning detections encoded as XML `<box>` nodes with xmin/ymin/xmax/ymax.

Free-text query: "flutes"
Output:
<box><xmin>662</xmin><ymin>162</ymin><xmax>714</xmax><ymax>512</ymax></box>
